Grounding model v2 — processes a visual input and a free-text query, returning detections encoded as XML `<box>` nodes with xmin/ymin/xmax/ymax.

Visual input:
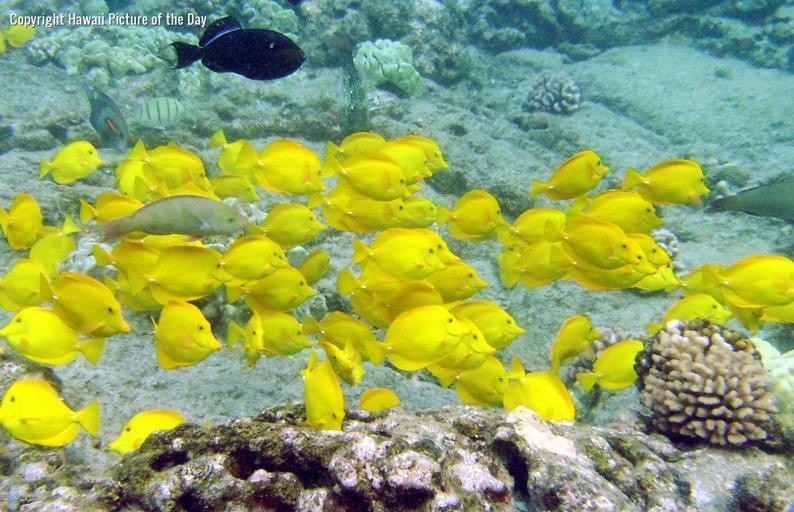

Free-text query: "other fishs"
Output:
<box><xmin>1</xmin><ymin>131</ymin><xmax>711</xmax><ymax>455</ymax></box>
